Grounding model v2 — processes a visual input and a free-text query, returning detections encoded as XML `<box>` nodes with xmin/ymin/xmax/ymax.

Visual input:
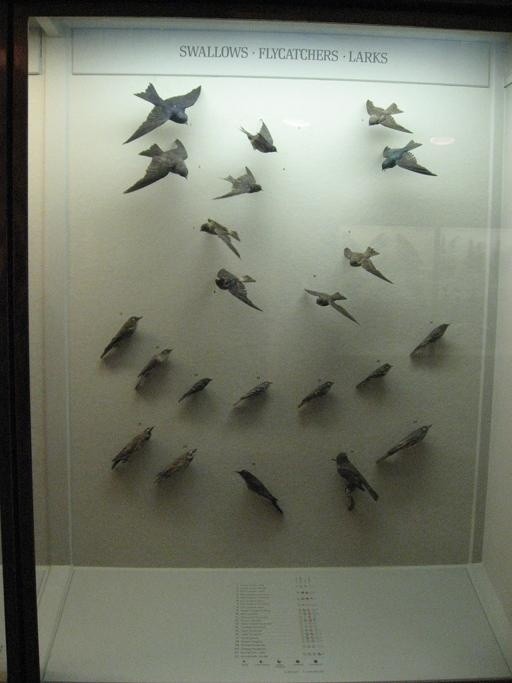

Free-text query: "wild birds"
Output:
<box><xmin>412</xmin><ymin>322</ymin><xmax>448</xmax><ymax>355</ymax></box>
<box><xmin>364</xmin><ymin>96</ymin><xmax>438</xmax><ymax>178</ymax></box>
<box><xmin>101</xmin><ymin>311</ymin><xmax>145</xmax><ymax>357</ymax></box>
<box><xmin>299</xmin><ymin>378</ymin><xmax>335</xmax><ymax>406</ymax></box>
<box><xmin>200</xmin><ymin>216</ymin><xmax>260</xmax><ymax>309</ymax></box>
<box><xmin>209</xmin><ymin>115</ymin><xmax>278</xmax><ymax>201</ymax></box>
<box><xmin>181</xmin><ymin>377</ymin><xmax>216</xmax><ymax>402</ymax></box>
<box><xmin>233</xmin><ymin>381</ymin><xmax>275</xmax><ymax>407</ymax></box>
<box><xmin>110</xmin><ymin>425</ymin><xmax>196</xmax><ymax>485</ymax></box>
<box><xmin>122</xmin><ymin>81</ymin><xmax>202</xmax><ymax>196</ymax></box>
<box><xmin>236</xmin><ymin>468</ymin><xmax>283</xmax><ymax>515</ymax></box>
<box><xmin>358</xmin><ymin>362</ymin><xmax>393</xmax><ymax>393</ymax></box>
<box><xmin>344</xmin><ymin>245</ymin><xmax>394</xmax><ymax>285</ymax></box>
<box><xmin>304</xmin><ymin>287</ymin><xmax>360</xmax><ymax>326</ymax></box>
<box><xmin>374</xmin><ymin>424</ymin><xmax>432</xmax><ymax>465</ymax></box>
<box><xmin>132</xmin><ymin>348</ymin><xmax>180</xmax><ymax>390</ymax></box>
<box><xmin>332</xmin><ymin>451</ymin><xmax>378</xmax><ymax>503</ymax></box>
